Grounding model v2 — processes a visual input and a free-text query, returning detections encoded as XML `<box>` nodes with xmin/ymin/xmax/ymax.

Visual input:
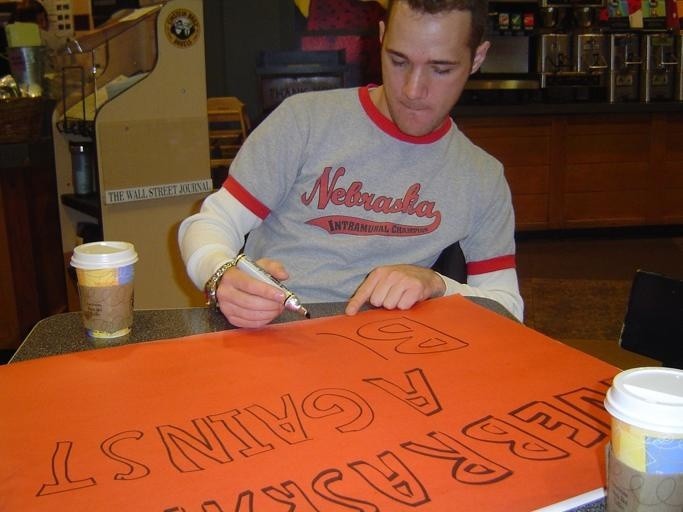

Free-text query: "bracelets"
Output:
<box><xmin>207</xmin><ymin>262</ymin><xmax>238</xmax><ymax>307</ymax></box>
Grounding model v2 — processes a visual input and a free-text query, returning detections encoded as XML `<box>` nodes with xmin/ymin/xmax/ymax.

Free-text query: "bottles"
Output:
<box><xmin>70</xmin><ymin>141</ymin><xmax>94</xmax><ymax>195</ymax></box>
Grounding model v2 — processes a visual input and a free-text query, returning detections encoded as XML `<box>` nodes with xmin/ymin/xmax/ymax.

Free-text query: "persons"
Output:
<box><xmin>175</xmin><ymin>0</ymin><xmax>527</xmax><ymax>328</ymax></box>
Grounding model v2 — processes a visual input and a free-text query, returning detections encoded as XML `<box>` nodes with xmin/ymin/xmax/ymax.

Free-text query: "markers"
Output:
<box><xmin>235</xmin><ymin>254</ymin><xmax>310</xmax><ymax>319</ymax></box>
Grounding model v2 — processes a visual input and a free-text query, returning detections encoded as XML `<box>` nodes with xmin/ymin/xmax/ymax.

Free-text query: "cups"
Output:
<box><xmin>70</xmin><ymin>241</ymin><xmax>139</xmax><ymax>339</ymax></box>
<box><xmin>604</xmin><ymin>367</ymin><xmax>683</xmax><ymax>512</ymax></box>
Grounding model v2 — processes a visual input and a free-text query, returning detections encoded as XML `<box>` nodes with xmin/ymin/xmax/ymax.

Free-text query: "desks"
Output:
<box><xmin>7</xmin><ymin>296</ymin><xmax>526</xmax><ymax>366</ymax></box>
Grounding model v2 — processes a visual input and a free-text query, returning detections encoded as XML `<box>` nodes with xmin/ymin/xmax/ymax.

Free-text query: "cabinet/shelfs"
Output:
<box><xmin>50</xmin><ymin>0</ymin><xmax>208</xmax><ymax>309</ymax></box>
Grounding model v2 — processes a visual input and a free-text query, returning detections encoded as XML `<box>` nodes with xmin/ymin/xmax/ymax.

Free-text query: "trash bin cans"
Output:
<box><xmin>256</xmin><ymin>46</ymin><xmax>352</xmax><ymax>120</ymax></box>
